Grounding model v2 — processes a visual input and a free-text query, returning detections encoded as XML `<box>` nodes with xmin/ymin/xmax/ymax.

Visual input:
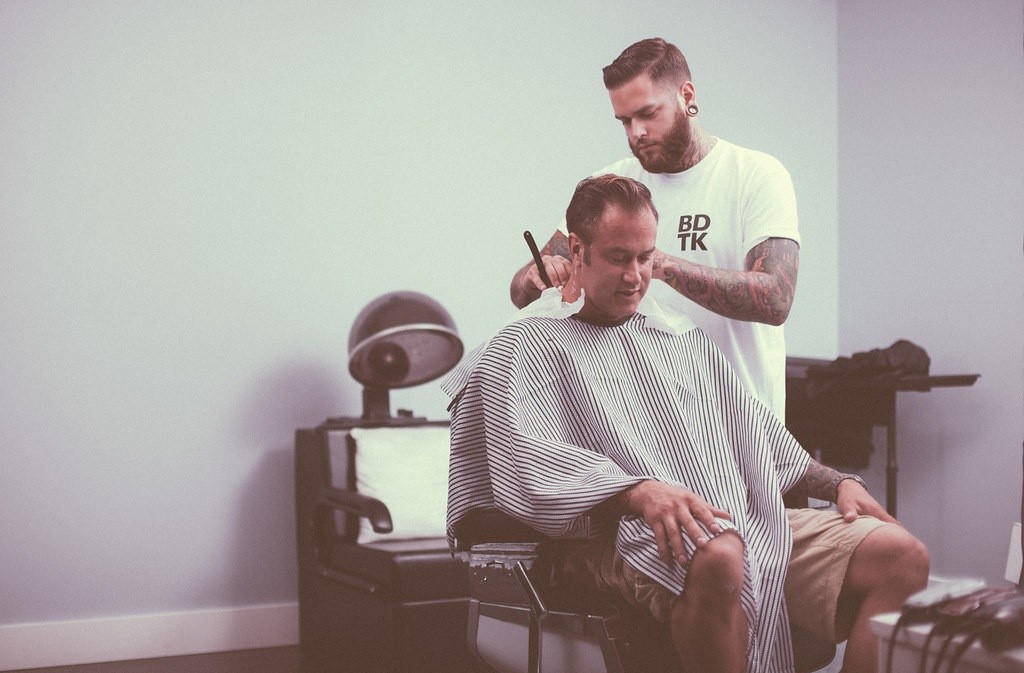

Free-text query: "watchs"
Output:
<box><xmin>833</xmin><ymin>473</ymin><xmax>868</xmax><ymax>504</ymax></box>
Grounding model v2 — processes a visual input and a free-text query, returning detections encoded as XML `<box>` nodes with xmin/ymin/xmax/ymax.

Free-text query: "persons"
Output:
<box><xmin>441</xmin><ymin>168</ymin><xmax>931</xmax><ymax>673</ymax></box>
<box><xmin>509</xmin><ymin>35</ymin><xmax>800</xmax><ymax>434</ymax></box>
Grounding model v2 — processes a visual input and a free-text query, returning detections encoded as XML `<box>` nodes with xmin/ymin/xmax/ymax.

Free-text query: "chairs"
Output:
<box><xmin>468</xmin><ymin>510</ymin><xmax>837</xmax><ymax>673</ymax></box>
<box><xmin>297</xmin><ymin>422</ymin><xmax>490</xmax><ymax>672</ymax></box>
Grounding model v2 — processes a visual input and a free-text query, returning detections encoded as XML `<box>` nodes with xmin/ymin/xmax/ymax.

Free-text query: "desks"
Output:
<box><xmin>785</xmin><ymin>357</ymin><xmax>983</xmax><ymax>520</ymax></box>
<box><xmin>869</xmin><ymin>611</ymin><xmax>1024</xmax><ymax>673</ymax></box>
